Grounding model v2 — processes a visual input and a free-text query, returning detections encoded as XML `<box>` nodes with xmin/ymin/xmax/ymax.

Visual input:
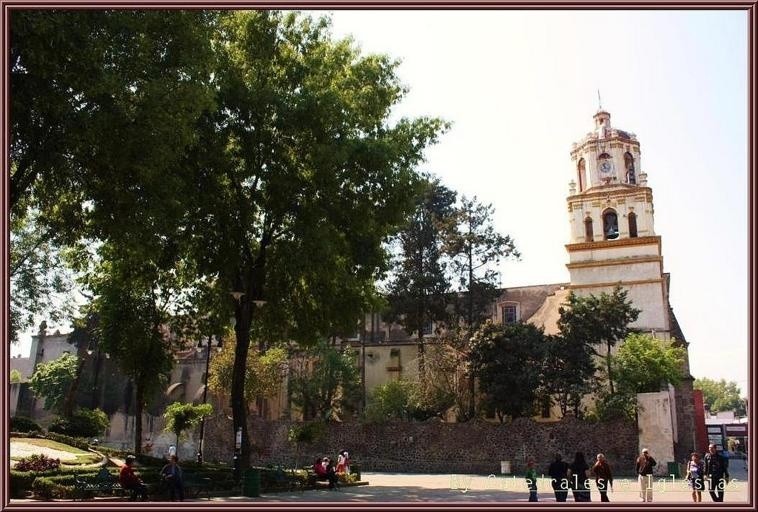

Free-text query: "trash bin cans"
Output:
<box><xmin>667</xmin><ymin>461</ymin><xmax>681</xmax><ymax>478</ymax></box>
<box><xmin>241</xmin><ymin>469</ymin><xmax>261</xmax><ymax>498</ymax></box>
<box><xmin>501</xmin><ymin>461</ymin><xmax>511</xmax><ymax>474</ymax></box>
<box><xmin>350</xmin><ymin>464</ymin><xmax>361</xmax><ymax>480</ymax></box>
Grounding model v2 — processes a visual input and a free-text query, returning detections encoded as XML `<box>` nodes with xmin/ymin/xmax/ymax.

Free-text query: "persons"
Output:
<box><xmin>526</xmin><ymin>461</ymin><xmax>538</xmax><ymax>502</ymax></box>
<box><xmin>119</xmin><ymin>455</ymin><xmax>148</xmax><ymax>501</ymax></box>
<box><xmin>548</xmin><ymin>453</ymin><xmax>572</xmax><ymax>502</ymax></box>
<box><xmin>159</xmin><ymin>457</ymin><xmax>184</xmax><ymax>502</ymax></box>
<box><xmin>592</xmin><ymin>453</ymin><xmax>612</xmax><ymax>502</ymax></box>
<box><xmin>634</xmin><ymin>448</ymin><xmax>657</xmax><ymax>502</ymax></box>
<box><xmin>169</xmin><ymin>444</ymin><xmax>177</xmax><ymax>456</ymax></box>
<box><xmin>569</xmin><ymin>451</ymin><xmax>591</xmax><ymax>501</ymax></box>
<box><xmin>703</xmin><ymin>442</ymin><xmax>729</xmax><ymax>503</ymax></box>
<box><xmin>313</xmin><ymin>449</ymin><xmax>352</xmax><ymax>484</ymax></box>
<box><xmin>685</xmin><ymin>452</ymin><xmax>705</xmax><ymax>502</ymax></box>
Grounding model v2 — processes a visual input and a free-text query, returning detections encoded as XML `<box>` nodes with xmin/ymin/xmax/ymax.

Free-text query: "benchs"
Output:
<box><xmin>75</xmin><ymin>480</ymin><xmax>164</xmax><ymax>498</ymax></box>
